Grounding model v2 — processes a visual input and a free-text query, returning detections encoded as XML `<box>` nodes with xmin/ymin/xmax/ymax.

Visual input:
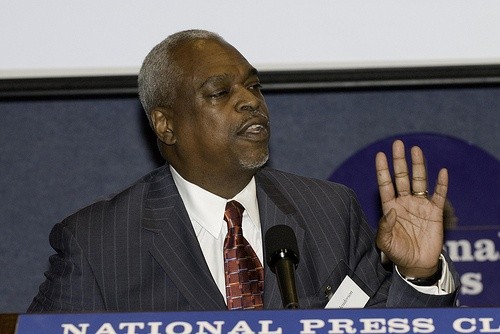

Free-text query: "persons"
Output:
<box><xmin>19</xmin><ymin>30</ymin><xmax>464</xmax><ymax>314</ymax></box>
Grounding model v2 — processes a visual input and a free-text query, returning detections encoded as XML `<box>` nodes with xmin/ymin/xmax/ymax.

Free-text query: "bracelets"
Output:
<box><xmin>398</xmin><ymin>256</ymin><xmax>443</xmax><ymax>287</ymax></box>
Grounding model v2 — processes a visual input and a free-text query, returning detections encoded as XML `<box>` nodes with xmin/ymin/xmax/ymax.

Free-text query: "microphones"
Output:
<box><xmin>265</xmin><ymin>225</ymin><xmax>300</xmax><ymax>309</ymax></box>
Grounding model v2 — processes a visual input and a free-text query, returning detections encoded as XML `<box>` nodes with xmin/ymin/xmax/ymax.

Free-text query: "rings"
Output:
<box><xmin>411</xmin><ymin>188</ymin><xmax>429</xmax><ymax>196</ymax></box>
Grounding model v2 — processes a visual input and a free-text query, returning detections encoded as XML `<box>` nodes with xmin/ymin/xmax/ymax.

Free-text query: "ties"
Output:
<box><xmin>222</xmin><ymin>200</ymin><xmax>265</xmax><ymax>310</ymax></box>
